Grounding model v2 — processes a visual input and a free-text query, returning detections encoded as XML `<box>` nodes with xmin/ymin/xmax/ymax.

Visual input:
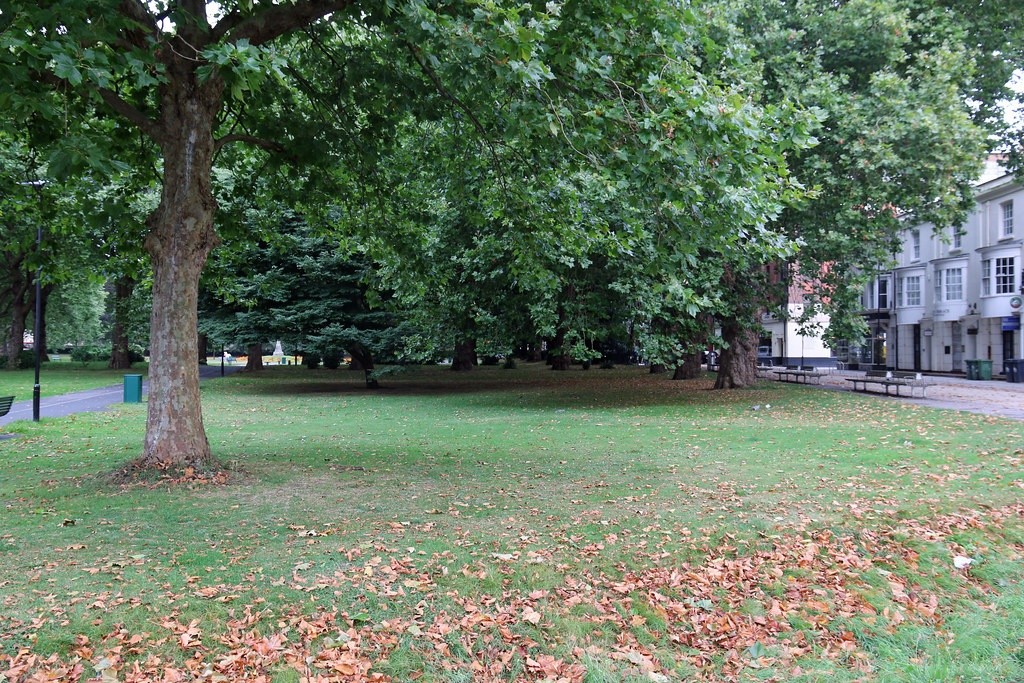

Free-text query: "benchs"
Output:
<box><xmin>845</xmin><ymin>377</ymin><xmax>937</xmax><ymax>398</ymax></box>
<box><xmin>711</xmin><ymin>365</ymin><xmax>720</xmax><ymax>371</ymax></box>
<box><xmin>757</xmin><ymin>367</ymin><xmax>773</xmax><ymax>372</ymax></box>
<box><xmin>0</xmin><ymin>395</ymin><xmax>16</xmax><ymax>417</ymax></box>
<box><xmin>774</xmin><ymin>371</ymin><xmax>828</xmax><ymax>385</ymax></box>
<box><xmin>52</xmin><ymin>354</ymin><xmax>61</xmax><ymax>361</ymax></box>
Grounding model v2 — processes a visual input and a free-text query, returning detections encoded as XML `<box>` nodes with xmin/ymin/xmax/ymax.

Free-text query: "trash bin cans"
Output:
<box><xmin>964</xmin><ymin>360</ymin><xmax>978</xmax><ymax>380</ymax></box>
<box><xmin>123</xmin><ymin>374</ymin><xmax>143</xmax><ymax>404</ymax></box>
<box><xmin>1002</xmin><ymin>358</ymin><xmax>1014</xmax><ymax>382</ymax></box>
<box><xmin>282</xmin><ymin>357</ymin><xmax>286</xmax><ymax>364</ymax></box>
<box><xmin>1012</xmin><ymin>359</ymin><xmax>1024</xmax><ymax>382</ymax></box>
<box><xmin>977</xmin><ymin>359</ymin><xmax>993</xmax><ymax>380</ymax></box>
<box><xmin>707</xmin><ymin>352</ymin><xmax>716</xmax><ymax>371</ymax></box>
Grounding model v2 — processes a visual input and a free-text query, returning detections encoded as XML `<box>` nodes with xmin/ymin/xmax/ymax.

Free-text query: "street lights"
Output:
<box><xmin>29</xmin><ymin>180</ymin><xmax>46</xmax><ymax>421</ymax></box>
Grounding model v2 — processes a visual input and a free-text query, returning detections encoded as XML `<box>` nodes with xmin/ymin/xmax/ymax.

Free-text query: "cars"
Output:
<box><xmin>701</xmin><ymin>350</ymin><xmax>720</xmax><ymax>364</ymax></box>
